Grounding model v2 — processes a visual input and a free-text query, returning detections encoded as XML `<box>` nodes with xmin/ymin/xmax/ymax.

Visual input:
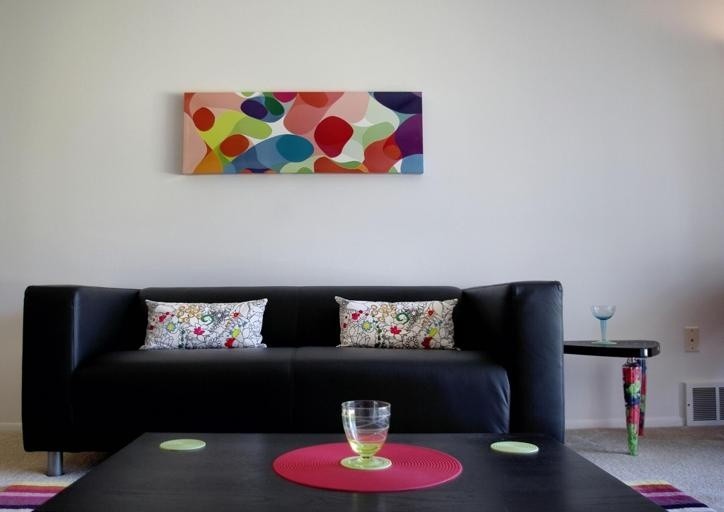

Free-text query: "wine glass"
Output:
<box><xmin>590</xmin><ymin>305</ymin><xmax>617</xmax><ymax>345</ymax></box>
<box><xmin>339</xmin><ymin>400</ymin><xmax>392</xmax><ymax>471</ymax></box>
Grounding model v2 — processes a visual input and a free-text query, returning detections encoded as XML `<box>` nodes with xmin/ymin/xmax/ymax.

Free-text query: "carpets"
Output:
<box><xmin>0</xmin><ymin>481</ymin><xmax>719</xmax><ymax>512</ymax></box>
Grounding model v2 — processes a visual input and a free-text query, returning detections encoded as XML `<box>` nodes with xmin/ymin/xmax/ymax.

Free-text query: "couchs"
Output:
<box><xmin>21</xmin><ymin>280</ymin><xmax>565</xmax><ymax>478</ymax></box>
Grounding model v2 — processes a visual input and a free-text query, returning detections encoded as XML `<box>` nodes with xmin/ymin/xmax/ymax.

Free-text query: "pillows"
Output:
<box><xmin>334</xmin><ymin>296</ymin><xmax>459</xmax><ymax>350</ymax></box>
<box><xmin>139</xmin><ymin>298</ymin><xmax>269</xmax><ymax>350</ymax></box>
